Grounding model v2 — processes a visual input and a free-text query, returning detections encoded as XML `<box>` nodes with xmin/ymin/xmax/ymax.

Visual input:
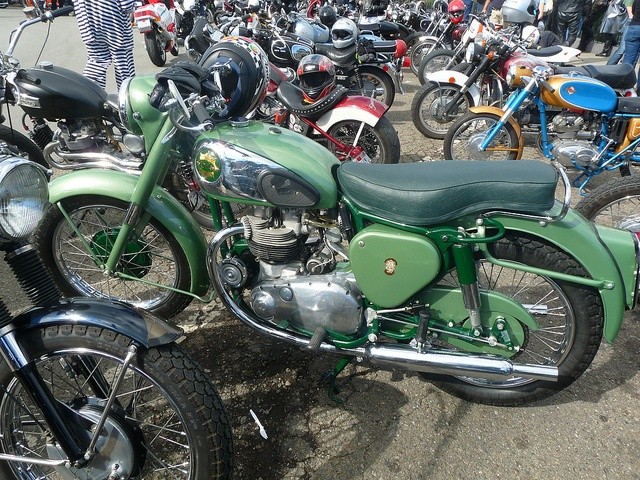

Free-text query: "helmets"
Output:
<box><xmin>394</xmin><ymin>39</ymin><xmax>407</xmax><ymax>59</ymax></box>
<box><xmin>319</xmin><ymin>6</ymin><xmax>336</xmax><ymax>26</ymax></box>
<box><xmin>297</xmin><ymin>54</ymin><xmax>336</xmax><ymax>103</ymax></box>
<box><xmin>448</xmin><ymin>0</ymin><xmax>464</xmax><ymax>24</ymax></box>
<box><xmin>331</xmin><ymin>18</ymin><xmax>357</xmax><ymax>49</ymax></box>
<box><xmin>522</xmin><ymin>25</ymin><xmax>540</xmax><ymax>44</ymax></box>
<box><xmin>502</xmin><ymin>0</ymin><xmax>535</xmax><ymax>24</ymax></box>
<box><xmin>199</xmin><ymin>36</ymin><xmax>269</xmax><ymax>118</ymax></box>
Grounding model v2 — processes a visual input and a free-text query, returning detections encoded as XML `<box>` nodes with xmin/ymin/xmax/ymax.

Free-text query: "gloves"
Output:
<box><xmin>155</xmin><ymin>61</ymin><xmax>210</xmax><ymax>94</ymax></box>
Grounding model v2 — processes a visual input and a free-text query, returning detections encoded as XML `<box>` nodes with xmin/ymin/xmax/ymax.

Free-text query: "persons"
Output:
<box><xmin>461</xmin><ymin>0</ymin><xmax>640</xmax><ymax>95</ymax></box>
<box><xmin>51</xmin><ymin>0</ymin><xmax>63</xmax><ymax>10</ymax></box>
<box><xmin>70</xmin><ymin>0</ymin><xmax>137</xmax><ymax>95</ymax></box>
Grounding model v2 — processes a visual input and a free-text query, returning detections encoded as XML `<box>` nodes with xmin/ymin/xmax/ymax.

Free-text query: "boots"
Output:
<box><xmin>595</xmin><ymin>36</ymin><xmax>613</xmax><ymax>57</ymax></box>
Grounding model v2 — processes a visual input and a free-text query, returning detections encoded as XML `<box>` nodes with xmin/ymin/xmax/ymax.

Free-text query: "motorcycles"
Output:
<box><xmin>190</xmin><ymin>6</ymin><xmax>400</xmax><ymax>166</ymax></box>
<box><xmin>1</xmin><ymin>0</ymin><xmax>234</xmax><ymax>479</ymax></box>
<box><xmin>412</xmin><ymin>20</ymin><xmax>636</xmax><ymax>139</ymax></box>
<box><xmin>418</xmin><ymin>11</ymin><xmax>504</xmax><ymax>86</ymax></box>
<box><xmin>37</xmin><ymin>36</ymin><xmax>639</xmax><ymax>406</ymax></box>
<box><xmin>2</xmin><ymin>10</ymin><xmax>140</xmax><ymax>175</ymax></box>
<box><xmin>133</xmin><ymin>0</ymin><xmax>179</xmax><ymax>67</ymax></box>
<box><xmin>277</xmin><ymin>8</ymin><xmax>407</xmax><ymax>109</ymax></box>
<box><xmin>409</xmin><ymin>0</ymin><xmax>481</xmax><ymax>77</ymax></box>
<box><xmin>443</xmin><ymin>62</ymin><xmax>638</xmax><ymax>196</ymax></box>
